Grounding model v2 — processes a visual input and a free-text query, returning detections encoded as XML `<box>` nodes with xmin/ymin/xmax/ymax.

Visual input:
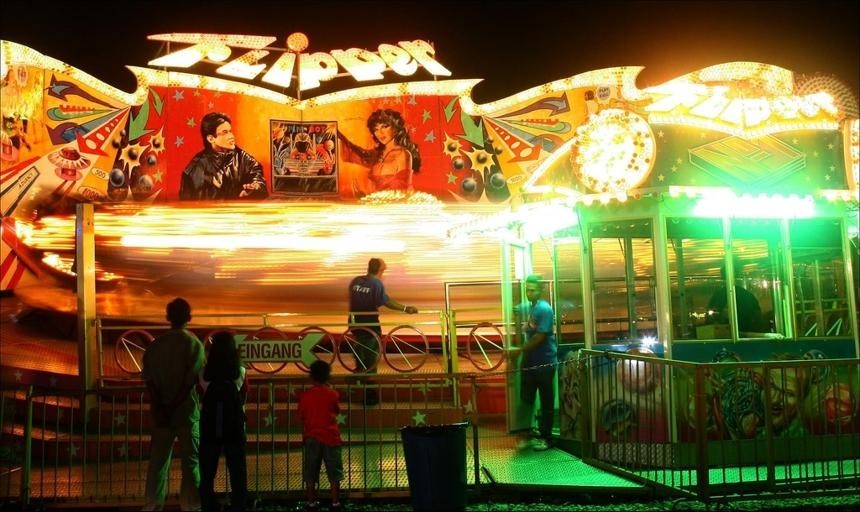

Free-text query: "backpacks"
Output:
<box><xmin>199</xmin><ymin>378</ymin><xmax>244</xmax><ymax>444</ymax></box>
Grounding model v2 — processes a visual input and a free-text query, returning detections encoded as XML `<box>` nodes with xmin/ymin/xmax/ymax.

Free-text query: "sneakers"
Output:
<box><xmin>534</xmin><ymin>438</ymin><xmax>549</xmax><ymax>452</ymax></box>
<box><xmin>330</xmin><ymin>504</ymin><xmax>341</xmax><ymax>511</ymax></box>
<box><xmin>305</xmin><ymin>503</ymin><xmax>320</xmax><ymax>510</ymax></box>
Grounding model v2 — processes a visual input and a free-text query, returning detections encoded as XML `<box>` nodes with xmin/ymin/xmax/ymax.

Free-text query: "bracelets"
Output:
<box><xmin>401</xmin><ymin>303</ymin><xmax>406</xmax><ymax>312</ymax></box>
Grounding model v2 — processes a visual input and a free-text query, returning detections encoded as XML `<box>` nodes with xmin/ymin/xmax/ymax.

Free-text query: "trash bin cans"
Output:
<box><xmin>399</xmin><ymin>422</ymin><xmax>468</xmax><ymax>512</ymax></box>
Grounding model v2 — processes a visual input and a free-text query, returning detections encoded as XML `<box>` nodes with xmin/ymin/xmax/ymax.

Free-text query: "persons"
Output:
<box><xmin>336</xmin><ymin>106</ymin><xmax>420</xmax><ymax>201</ymax></box>
<box><xmin>140</xmin><ymin>298</ymin><xmax>204</xmax><ymax>511</ymax></box>
<box><xmin>704</xmin><ymin>256</ymin><xmax>766</xmax><ymax>339</ymax></box>
<box><xmin>344</xmin><ymin>255</ymin><xmax>418</xmax><ymax>406</ymax></box>
<box><xmin>503</xmin><ymin>272</ymin><xmax>561</xmax><ymax>450</ymax></box>
<box><xmin>289</xmin><ymin>360</ymin><xmax>352</xmax><ymax>508</ymax></box>
<box><xmin>196</xmin><ymin>331</ymin><xmax>250</xmax><ymax>509</ymax></box>
<box><xmin>180</xmin><ymin>109</ymin><xmax>269</xmax><ymax>201</ymax></box>
<box><xmin>750</xmin><ymin>350</ymin><xmax>816</xmax><ymax>439</ymax></box>
